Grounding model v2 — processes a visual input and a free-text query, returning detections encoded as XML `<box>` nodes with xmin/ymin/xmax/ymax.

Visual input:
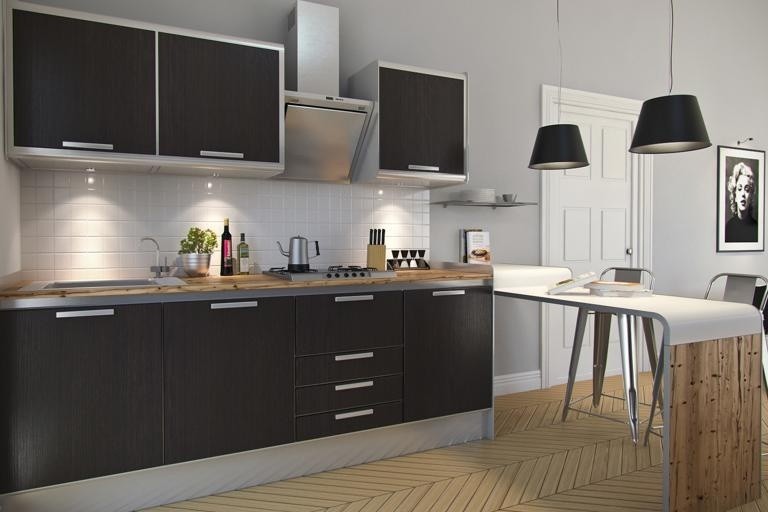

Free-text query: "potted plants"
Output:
<box><xmin>177</xmin><ymin>227</ymin><xmax>218</xmax><ymax>277</ymax></box>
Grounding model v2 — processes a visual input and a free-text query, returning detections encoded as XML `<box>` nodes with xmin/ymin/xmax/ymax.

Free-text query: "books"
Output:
<box><xmin>589</xmin><ymin>289</ymin><xmax>655</xmax><ymax>297</ymax></box>
<box><xmin>458</xmin><ymin>228</ymin><xmax>484</xmax><ymax>265</ymax></box>
<box><xmin>584</xmin><ymin>281</ymin><xmax>644</xmax><ymax>291</ymax></box>
<box><xmin>546</xmin><ymin>271</ymin><xmax>597</xmax><ymax>296</ymax></box>
<box><xmin>466</xmin><ymin>231</ymin><xmax>492</xmax><ymax>266</ymax></box>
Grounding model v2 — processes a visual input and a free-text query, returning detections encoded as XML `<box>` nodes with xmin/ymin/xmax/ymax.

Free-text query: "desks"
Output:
<box><xmin>493</xmin><ymin>264</ymin><xmax>763</xmax><ymax>512</ymax></box>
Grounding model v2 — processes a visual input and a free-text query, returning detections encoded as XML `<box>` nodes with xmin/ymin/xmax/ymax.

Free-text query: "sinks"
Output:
<box><xmin>39</xmin><ymin>278</ymin><xmax>163</xmax><ymax>291</ymax></box>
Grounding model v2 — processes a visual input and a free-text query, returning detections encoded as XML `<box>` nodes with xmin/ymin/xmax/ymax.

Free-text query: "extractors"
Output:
<box><xmin>278</xmin><ymin>0</ymin><xmax>376</xmax><ymax>187</ymax></box>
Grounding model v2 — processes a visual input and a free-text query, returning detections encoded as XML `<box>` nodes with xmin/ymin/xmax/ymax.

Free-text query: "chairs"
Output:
<box><xmin>561</xmin><ymin>267</ymin><xmax>664</xmax><ymax>445</ymax></box>
<box><xmin>643</xmin><ymin>273</ymin><xmax>768</xmax><ymax>457</ymax></box>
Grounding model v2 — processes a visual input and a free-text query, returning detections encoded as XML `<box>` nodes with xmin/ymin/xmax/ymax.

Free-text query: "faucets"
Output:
<box><xmin>136</xmin><ymin>237</ymin><xmax>169</xmax><ymax>277</ymax></box>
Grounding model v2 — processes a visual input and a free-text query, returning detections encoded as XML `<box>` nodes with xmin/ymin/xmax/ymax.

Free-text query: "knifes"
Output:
<box><xmin>368</xmin><ymin>228</ymin><xmax>385</xmax><ymax>245</ymax></box>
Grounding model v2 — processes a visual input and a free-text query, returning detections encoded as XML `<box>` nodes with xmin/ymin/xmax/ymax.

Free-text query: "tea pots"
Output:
<box><xmin>277</xmin><ymin>234</ymin><xmax>320</xmax><ymax>271</ymax></box>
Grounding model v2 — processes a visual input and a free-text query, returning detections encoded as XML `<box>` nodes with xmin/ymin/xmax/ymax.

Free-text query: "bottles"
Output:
<box><xmin>220</xmin><ymin>217</ymin><xmax>234</xmax><ymax>276</ymax></box>
<box><xmin>418</xmin><ymin>249</ymin><xmax>426</xmax><ymax>267</ymax></box>
<box><xmin>410</xmin><ymin>250</ymin><xmax>418</xmax><ymax>268</ymax></box>
<box><xmin>391</xmin><ymin>249</ymin><xmax>400</xmax><ymax>268</ymax></box>
<box><xmin>237</xmin><ymin>232</ymin><xmax>249</xmax><ymax>274</ymax></box>
<box><xmin>401</xmin><ymin>250</ymin><xmax>408</xmax><ymax>267</ymax></box>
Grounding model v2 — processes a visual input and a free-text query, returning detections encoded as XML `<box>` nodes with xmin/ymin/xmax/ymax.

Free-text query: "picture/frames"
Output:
<box><xmin>715</xmin><ymin>144</ymin><xmax>766</xmax><ymax>253</ymax></box>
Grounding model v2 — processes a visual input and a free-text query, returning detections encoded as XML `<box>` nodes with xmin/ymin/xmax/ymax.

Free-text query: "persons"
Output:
<box><xmin>724</xmin><ymin>162</ymin><xmax>757</xmax><ymax>242</ymax></box>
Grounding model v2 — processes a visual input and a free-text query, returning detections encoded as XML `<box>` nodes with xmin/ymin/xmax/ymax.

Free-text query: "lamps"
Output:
<box><xmin>629</xmin><ymin>0</ymin><xmax>713</xmax><ymax>155</ymax></box>
<box><xmin>527</xmin><ymin>0</ymin><xmax>590</xmax><ymax>171</ymax></box>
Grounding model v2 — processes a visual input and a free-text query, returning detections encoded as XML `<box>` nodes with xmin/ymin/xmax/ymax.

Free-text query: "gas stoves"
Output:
<box><xmin>261</xmin><ymin>264</ymin><xmax>398</xmax><ymax>280</ymax></box>
